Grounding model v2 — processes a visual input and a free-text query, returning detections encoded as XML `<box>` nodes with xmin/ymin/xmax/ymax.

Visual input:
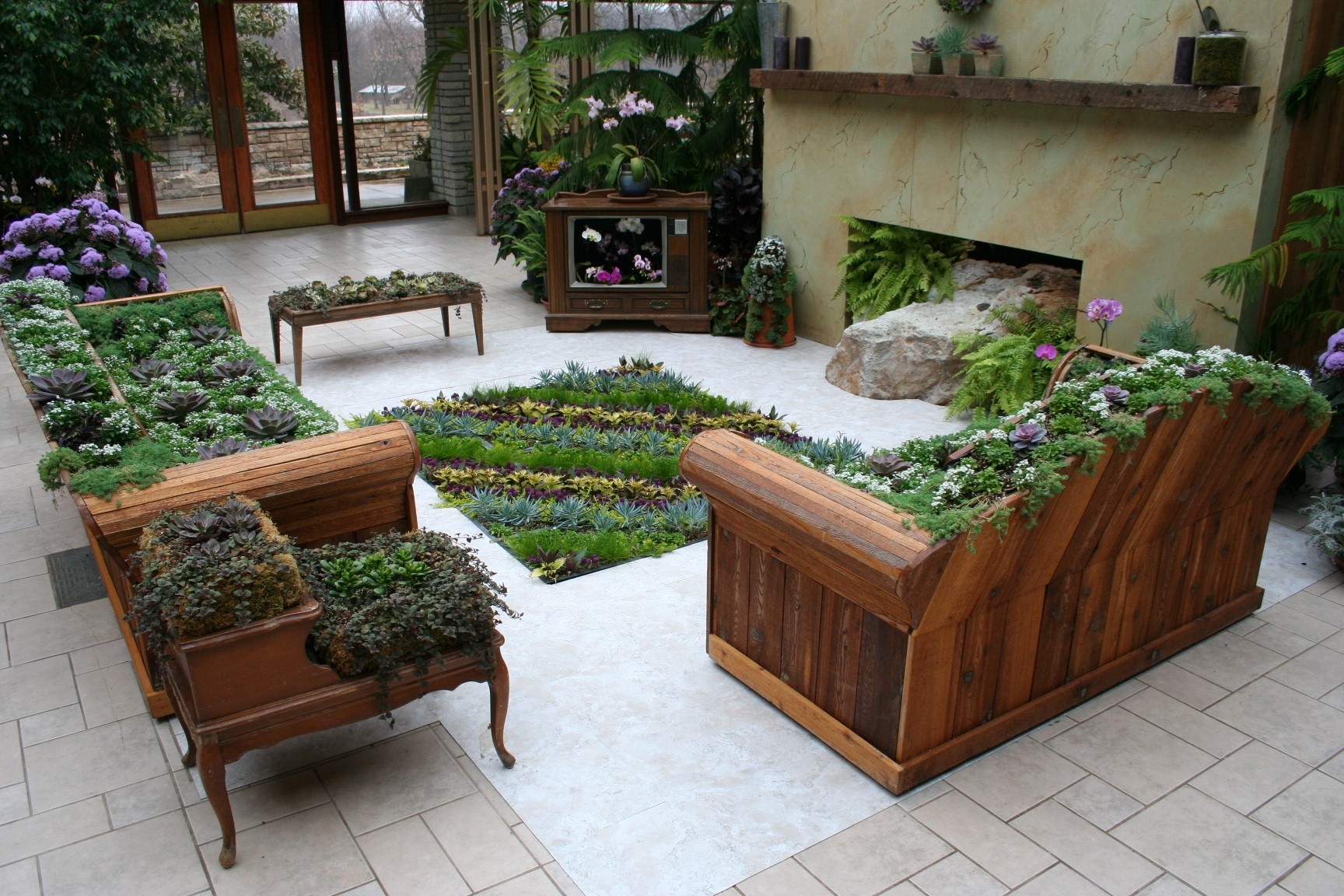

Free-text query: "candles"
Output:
<box><xmin>1173</xmin><ymin>36</ymin><xmax>1196</xmax><ymax>85</ymax></box>
<box><xmin>794</xmin><ymin>36</ymin><xmax>811</xmax><ymax>71</ymax></box>
<box><xmin>775</xmin><ymin>35</ymin><xmax>789</xmax><ymax>70</ymax></box>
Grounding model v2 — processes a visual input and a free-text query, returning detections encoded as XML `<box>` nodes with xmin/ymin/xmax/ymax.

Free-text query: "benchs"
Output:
<box><xmin>677</xmin><ymin>373</ymin><xmax>1332</xmax><ymax>798</ymax></box>
<box><xmin>0</xmin><ymin>285</ymin><xmax>422</xmax><ymax>721</ymax></box>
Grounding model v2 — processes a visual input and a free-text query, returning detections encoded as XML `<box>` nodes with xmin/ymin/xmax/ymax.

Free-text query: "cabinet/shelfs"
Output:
<box><xmin>540</xmin><ymin>188</ymin><xmax>712</xmax><ymax>333</ymax></box>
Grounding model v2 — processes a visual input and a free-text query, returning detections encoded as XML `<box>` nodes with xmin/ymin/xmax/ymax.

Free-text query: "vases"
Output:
<box><xmin>1192</xmin><ymin>28</ymin><xmax>1250</xmax><ymax>88</ymax></box>
<box><xmin>539</xmin><ymin>271</ymin><xmax>548</xmax><ymax>311</ymax></box>
<box><xmin>744</xmin><ymin>294</ymin><xmax>798</xmax><ymax>348</ymax></box>
<box><xmin>618</xmin><ymin>169</ymin><xmax>652</xmax><ymax>197</ymax></box>
<box><xmin>526</xmin><ymin>254</ymin><xmax>547</xmax><ymax>285</ymax></box>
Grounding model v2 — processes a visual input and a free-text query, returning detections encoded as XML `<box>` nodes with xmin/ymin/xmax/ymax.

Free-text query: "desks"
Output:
<box><xmin>267</xmin><ymin>278</ymin><xmax>486</xmax><ymax>386</ymax></box>
<box><xmin>157</xmin><ymin>598</ymin><xmax>515</xmax><ymax>871</ymax></box>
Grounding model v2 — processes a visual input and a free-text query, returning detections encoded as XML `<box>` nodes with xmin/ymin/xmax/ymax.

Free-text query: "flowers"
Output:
<box><xmin>747</xmin><ymin>297</ymin><xmax>1344</xmax><ymax>549</ymax></box>
<box><xmin>479</xmin><ymin>82</ymin><xmax>795</xmax><ymax>346</ymax></box>
<box><xmin>0</xmin><ymin>178</ymin><xmax>339</xmax><ymax>509</ymax></box>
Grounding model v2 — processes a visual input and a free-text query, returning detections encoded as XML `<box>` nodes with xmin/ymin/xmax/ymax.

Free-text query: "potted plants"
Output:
<box><xmin>406</xmin><ymin>133</ymin><xmax>431</xmax><ymax>178</ymax></box>
<box><xmin>911</xmin><ymin>24</ymin><xmax>1005</xmax><ymax>77</ymax></box>
<box><xmin>124</xmin><ymin>497</ymin><xmax>344</xmax><ymax>724</ymax></box>
